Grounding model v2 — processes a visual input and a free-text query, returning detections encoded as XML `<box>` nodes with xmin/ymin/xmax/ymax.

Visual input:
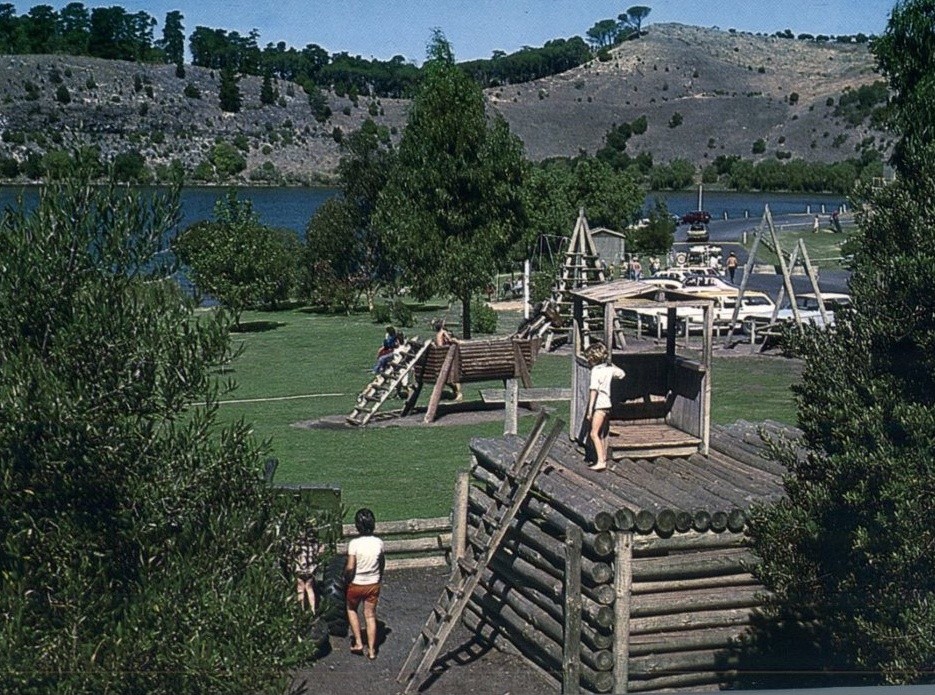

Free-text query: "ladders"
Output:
<box><xmin>346</xmin><ymin>335</ymin><xmax>431</xmax><ymax>425</ymax></box>
<box><xmin>397</xmin><ymin>411</ymin><xmax>566</xmax><ymax>695</ymax></box>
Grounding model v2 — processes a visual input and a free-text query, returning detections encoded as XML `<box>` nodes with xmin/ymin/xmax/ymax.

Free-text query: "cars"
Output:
<box><xmin>612</xmin><ymin>210</ymin><xmax>777</xmax><ymax>339</ymax></box>
<box><xmin>743</xmin><ymin>292</ymin><xmax>854</xmax><ymax>345</ymax></box>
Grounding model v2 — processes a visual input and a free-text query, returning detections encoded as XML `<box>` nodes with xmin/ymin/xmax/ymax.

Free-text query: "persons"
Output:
<box><xmin>344</xmin><ymin>509</ymin><xmax>385</xmax><ymax>660</ymax></box>
<box><xmin>290</xmin><ymin>522</ymin><xmax>320</xmax><ymax>616</ymax></box>
<box><xmin>620</xmin><ymin>257</ymin><xmax>641</xmax><ymax>281</ymax></box>
<box><xmin>434</xmin><ymin>320</ymin><xmax>463</xmax><ymax>401</ymax></box>
<box><xmin>813</xmin><ymin>214</ymin><xmax>818</xmax><ymax>233</ymax></box>
<box><xmin>710</xmin><ymin>251</ymin><xmax>724</xmax><ymax>278</ymax></box>
<box><xmin>648</xmin><ymin>254</ymin><xmax>660</xmax><ymax>276</ymax></box>
<box><xmin>503</xmin><ymin>275</ymin><xmax>523</xmax><ymax>297</ymax></box>
<box><xmin>371</xmin><ymin>326</ymin><xmax>408</xmax><ymax>400</ymax></box>
<box><xmin>828</xmin><ymin>210</ymin><xmax>842</xmax><ymax>233</ymax></box>
<box><xmin>600</xmin><ymin>259</ymin><xmax>615</xmax><ymax>281</ymax></box>
<box><xmin>583</xmin><ymin>342</ymin><xmax>626</xmax><ymax>471</ymax></box>
<box><xmin>727</xmin><ymin>251</ymin><xmax>737</xmax><ymax>284</ymax></box>
<box><xmin>486</xmin><ymin>278</ymin><xmax>496</xmax><ymax>302</ymax></box>
